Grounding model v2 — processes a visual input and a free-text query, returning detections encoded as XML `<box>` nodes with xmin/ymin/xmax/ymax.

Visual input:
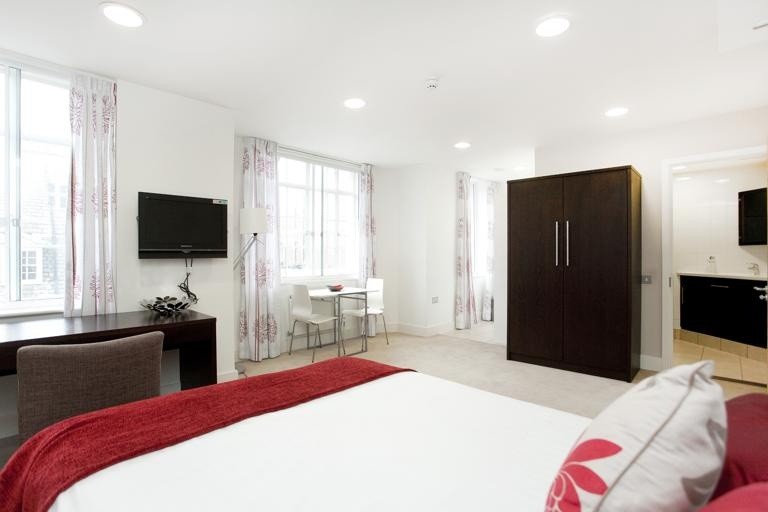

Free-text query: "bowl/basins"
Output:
<box><xmin>326</xmin><ymin>285</ymin><xmax>344</xmax><ymax>292</ymax></box>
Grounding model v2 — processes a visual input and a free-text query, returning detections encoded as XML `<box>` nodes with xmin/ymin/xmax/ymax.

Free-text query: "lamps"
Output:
<box><xmin>232</xmin><ymin>207</ymin><xmax>268</xmax><ymax>271</ymax></box>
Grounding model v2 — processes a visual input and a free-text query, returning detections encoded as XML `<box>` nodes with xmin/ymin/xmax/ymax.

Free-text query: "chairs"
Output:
<box><xmin>16</xmin><ymin>330</ymin><xmax>165</xmax><ymax>448</ymax></box>
<box><xmin>280</xmin><ymin>270</ymin><xmax>389</xmax><ymax>364</ymax></box>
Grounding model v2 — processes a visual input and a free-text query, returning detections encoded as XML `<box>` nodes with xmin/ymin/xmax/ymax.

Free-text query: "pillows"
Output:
<box><xmin>545</xmin><ymin>358</ymin><xmax>727</xmax><ymax>512</ymax></box>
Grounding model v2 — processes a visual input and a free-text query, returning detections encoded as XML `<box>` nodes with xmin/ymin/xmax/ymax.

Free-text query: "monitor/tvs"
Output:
<box><xmin>138</xmin><ymin>191</ymin><xmax>228</xmax><ymax>259</ymax></box>
<box><xmin>738</xmin><ymin>187</ymin><xmax>768</xmax><ymax>246</ymax></box>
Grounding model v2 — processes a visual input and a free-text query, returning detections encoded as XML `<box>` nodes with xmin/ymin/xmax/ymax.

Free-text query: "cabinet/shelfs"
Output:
<box><xmin>506</xmin><ymin>164</ymin><xmax>642</xmax><ymax>384</ymax></box>
<box><xmin>680</xmin><ymin>275</ymin><xmax>767</xmax><ymax>350</ymax></box>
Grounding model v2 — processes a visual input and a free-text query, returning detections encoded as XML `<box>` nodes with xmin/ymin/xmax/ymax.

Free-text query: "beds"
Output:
<box><xmin>1</xmin><ymin>354</ymin><xmax>767</xmax><ymax>512</ymax></box>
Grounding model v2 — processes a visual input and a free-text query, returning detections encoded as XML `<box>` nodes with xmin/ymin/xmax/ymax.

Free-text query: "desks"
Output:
<box><xmin>0</xmin><ymin>308</ymin><xmax>217</xmax><ymax>392</ymax></box>
